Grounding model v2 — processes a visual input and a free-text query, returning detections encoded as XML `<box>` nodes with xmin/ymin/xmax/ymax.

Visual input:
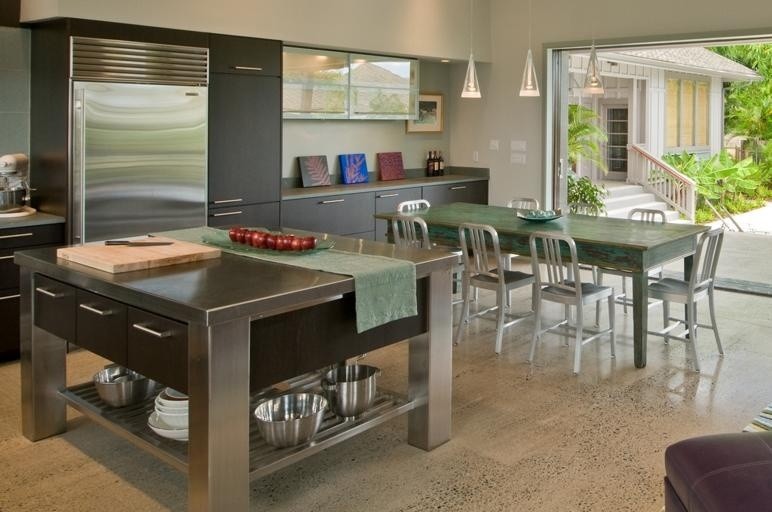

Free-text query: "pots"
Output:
<box><xmin>323</xmin><ymin>364</ymin><xmax>383</xmax><ymax>417</ymax></box>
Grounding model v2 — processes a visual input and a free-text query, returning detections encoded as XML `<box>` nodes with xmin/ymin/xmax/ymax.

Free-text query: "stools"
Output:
<box><xmin>662</xmin><ymin>430</ymin><xmax>772</xmax><ymax>512</ymax></box>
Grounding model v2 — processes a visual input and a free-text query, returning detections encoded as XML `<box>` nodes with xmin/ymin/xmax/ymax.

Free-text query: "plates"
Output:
<box><xmin>516</xmin><ymin>209</ymin><xmax>562</xmax><ymax>223</ymax></box>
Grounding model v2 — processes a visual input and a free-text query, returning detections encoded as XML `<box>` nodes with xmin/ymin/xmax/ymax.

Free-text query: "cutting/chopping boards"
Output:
<box><xmin>57</xmin><ymin>235</ymin><xmax>220</xmax><ymax>274</ymax></box>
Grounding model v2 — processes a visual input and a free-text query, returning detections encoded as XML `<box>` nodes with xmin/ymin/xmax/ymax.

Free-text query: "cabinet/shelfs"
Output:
<box><xmin>209</xmin><ymin>200</ymin><xmax>280</xmax><ymax>234</ymax></box>
<box><xmin>282</xmin><ymin>45</ymin><xmax>422</xmax><ymax>122</ymax></box>
<box><xmin>0</xmin><ymin>223</ymin><xmax>65</xmax><ymax>367</ymax></box>
<box><xmin>376</xmin><ymin>187</ymin><xmax>423</xmax><ymax>244</ymax></box>
<box><xmin>208</xmin><ymin>75</ymin><xmax>281</xmax><ymax>210</ymax></box>
<box><xmin>210</xmin><ymin>32</ymin><xmax>282</xmax><ymax>78</ymax></box>
<box><xmin>423</xmin><ymin>181</ymin><xmax>489</xmax><ymax>205</ymax></box>
<box><xmin>283</xmin><ymin>192</ymin><xmax>376</xmax><ymax>241</ymax></box>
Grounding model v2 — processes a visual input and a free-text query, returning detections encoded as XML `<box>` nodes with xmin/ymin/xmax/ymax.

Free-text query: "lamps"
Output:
<box><xmin>460</xmin><ymin>0</ymin><xmax>481</xmax><ymax>98</ymax></box>
<box><xmin>581</xmin><ymin>1</ymin><xmax>608</xmax><ymax>95</ymax></box>
<box><xmin>518</xmin><ymin>0</ymin><xmax>542</xmax><ymax>97</ymax></box>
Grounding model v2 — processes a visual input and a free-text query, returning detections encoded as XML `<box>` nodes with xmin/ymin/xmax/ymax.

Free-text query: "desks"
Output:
<box><xmin>13</xmin><ymin>220</ymin><xmax>460</xmax><ymax>511</ymax></box>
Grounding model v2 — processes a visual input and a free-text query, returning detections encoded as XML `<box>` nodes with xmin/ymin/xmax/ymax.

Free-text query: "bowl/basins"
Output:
<box><xmin>147</xmin><ymin>387</ymin><xmax>188</xmax><ymax>442</ymax></box>
<box><xmin>255</xmin><ymin>392</ymin><xmax>328</xmax><ymax>446</ymax></box>
<box><xmin>94</xmin><ymin>366</ymin><xmax>158</xmax><ymax>406</ymax></box>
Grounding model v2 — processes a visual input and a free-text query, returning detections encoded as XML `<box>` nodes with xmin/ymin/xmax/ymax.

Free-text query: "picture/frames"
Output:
<box><xmin>405</xmin><ymin>93</ymin><xmax>444</xmax><ymax>133</ymax></box>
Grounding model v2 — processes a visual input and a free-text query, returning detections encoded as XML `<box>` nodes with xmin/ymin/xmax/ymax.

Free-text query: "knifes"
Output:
<box><xmin>106</xmin><ymin>238</ymin><xmax>172</xmax><ymax>248</ymax></box>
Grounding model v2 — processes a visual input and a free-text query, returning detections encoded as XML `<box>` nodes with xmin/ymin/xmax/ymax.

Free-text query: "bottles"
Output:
<box><xmin>425</xmin><ymin>151</ymin><xmax>445</xmax><ymax>178</ymax></box>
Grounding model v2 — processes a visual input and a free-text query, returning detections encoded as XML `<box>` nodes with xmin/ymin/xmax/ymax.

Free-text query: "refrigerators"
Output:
<box><xmin>67</xmin><ymin>80</ymin><xmax>210</xmax><ymax>246</ymax></box>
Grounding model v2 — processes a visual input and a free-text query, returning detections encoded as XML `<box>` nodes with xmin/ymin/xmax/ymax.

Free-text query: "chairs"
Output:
<box><xmin>391</xmin><ymin>215</ymin><xmax>472</xmax><ymax>327</ymax></box>
<box><xmin>397</xmin><ymin>199</ymin><xmax>478</xmax><ymax>301</ymax></box>
<box><xmin>565</xmin><ymin>202</ymin><xmax>606</xmax><ymax>326</ymax></box>
<box><xmin>594</xmin><ymin>208</ymin><xmax>667</xmax><ymax>328</ymax></box>
<box><xmin>472</xmin><ymin>197</ymin><xmax>542</xmax><ymax>307</ymax></box>
<box><xmin>647</xmin><ymin>229</ymin><xmax>724</xmax><ymax>372</ymax></box>
<box><xmin>453</xmin><ymin>222</ymin><xmax>538</xmax><ymax>355</ymax></box>
<box><xmin>526</xmin><ymin>230</ymin><xmax>616</xmax><ymax>374</ymax></box>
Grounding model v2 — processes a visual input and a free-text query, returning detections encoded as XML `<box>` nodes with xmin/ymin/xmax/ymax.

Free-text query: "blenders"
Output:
<box><xmin>0</xmin><ymin>154</ymin><xmax>35</xmax><ymax>218</ymax></box>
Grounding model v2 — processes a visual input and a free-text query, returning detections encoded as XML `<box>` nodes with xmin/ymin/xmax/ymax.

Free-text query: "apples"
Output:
<box><xmin>228</xmin><ymin>226</ymin><xmax>318</xmax><ymax>251</ymax></box>
